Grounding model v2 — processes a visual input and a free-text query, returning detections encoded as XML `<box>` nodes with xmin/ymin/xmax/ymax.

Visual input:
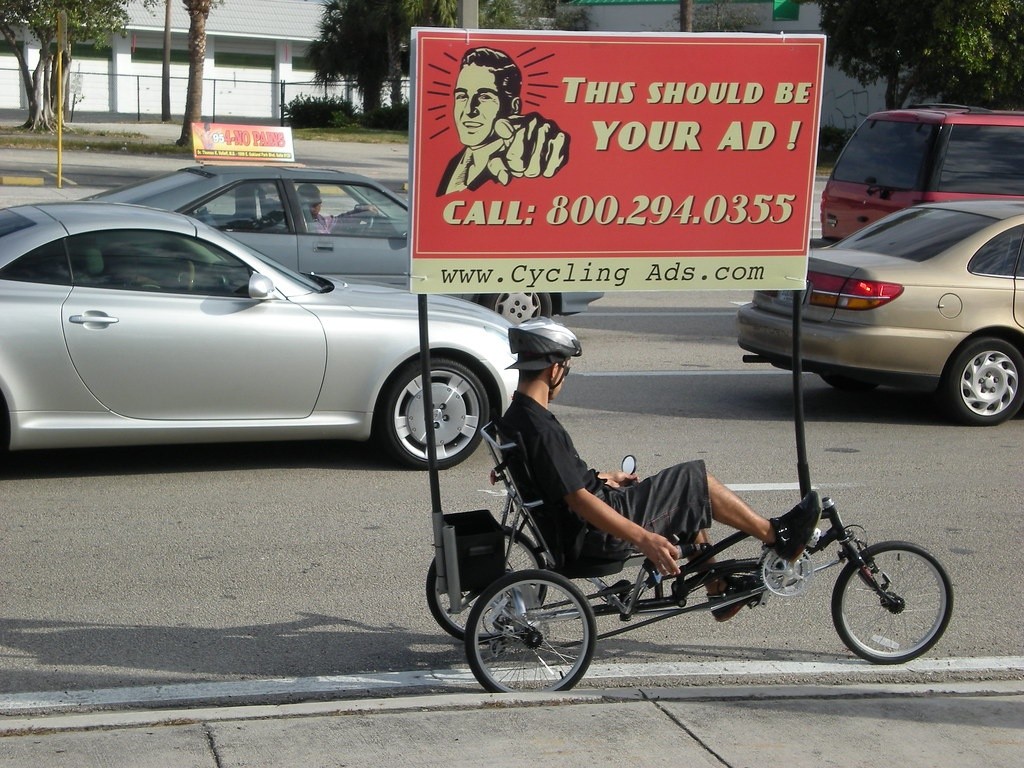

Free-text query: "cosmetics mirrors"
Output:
<box><xmin>621</xmin><ymin>455</ymin><xmax>636</xmax><ymax>475</ymax></box>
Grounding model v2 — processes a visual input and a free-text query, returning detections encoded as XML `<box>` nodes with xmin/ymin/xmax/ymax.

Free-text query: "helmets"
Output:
<box><xmin>509</xmin><ymin>319</ymin><xmax>583</xmax><ymax>356</ymax></box>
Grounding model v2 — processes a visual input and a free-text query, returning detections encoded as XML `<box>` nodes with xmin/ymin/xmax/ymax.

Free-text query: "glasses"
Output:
<box><xmin>559</xmin><ymin>364</ymin><xmax>571</xmax><ymax>376</ymax></box>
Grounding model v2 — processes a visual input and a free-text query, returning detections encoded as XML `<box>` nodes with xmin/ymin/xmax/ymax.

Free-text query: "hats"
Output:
<box><xmin>504</xmin><ymin>353</ymin><xmax>571</xmax><ymax>370</ymax></box>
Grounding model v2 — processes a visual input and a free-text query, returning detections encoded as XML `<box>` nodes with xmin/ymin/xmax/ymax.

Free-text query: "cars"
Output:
<box><xmin>0</xmin><ymin>199</ymin><xmax>520</xmax><ymax>471</ymax></box>
<box><xmin>735</xmin><ymin>202</ymin><xmax>1024</xmax><ymax>426</ymax></box>
<box><xmin>83</xmin><ymin>163</ymin><xmax>607</xmax><ymax>329</ymax></box>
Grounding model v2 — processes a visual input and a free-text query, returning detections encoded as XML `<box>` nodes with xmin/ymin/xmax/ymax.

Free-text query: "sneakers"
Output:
<box><xmin>762</xmin><ymin>491</ymin><xmax>822</xmax><ymax>563</ymax></box>
<box><xmin>706</xmin><ymin>576</ymin><xmax>761</xmax><ymax>621</ymax></box>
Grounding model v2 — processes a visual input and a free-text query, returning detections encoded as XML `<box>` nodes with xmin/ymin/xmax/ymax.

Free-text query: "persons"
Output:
<box><xmin>103</xmin><ymin>242</ymin><xmax>163</xmax><ymax>287</ymax></box>
<box><xmin>498</xmin><ymin>315</ymin><xmax>822</xmax><ymax>622</ymax></box>
<box><xmin>298</xmin><ymin>184</ymin><xmax>381</xmax><ymax>235</ymax></box>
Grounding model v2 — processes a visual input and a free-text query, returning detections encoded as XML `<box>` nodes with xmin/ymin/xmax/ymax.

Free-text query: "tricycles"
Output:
<box><xmin>407</xmin><ymin>293</ymin><xmax>954</xmax><ymax>695</ymax></box>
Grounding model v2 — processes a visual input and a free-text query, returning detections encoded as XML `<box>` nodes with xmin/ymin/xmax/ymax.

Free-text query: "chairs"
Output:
<box><xmin>78</xmin><ymin>248</ymin><xmax>103</xmax><ymax>280</ymax></box>
<box><xmin>478</xmin><ymin>421</ymin><xmax>647</xmax><ymax>580</ymax></box>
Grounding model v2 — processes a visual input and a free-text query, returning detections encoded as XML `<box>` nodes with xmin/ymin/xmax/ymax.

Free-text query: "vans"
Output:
<box><xmin>819</xmin><ymin>103</ymin><xmax>1024</xmax><ymax>243</ymax></box>
